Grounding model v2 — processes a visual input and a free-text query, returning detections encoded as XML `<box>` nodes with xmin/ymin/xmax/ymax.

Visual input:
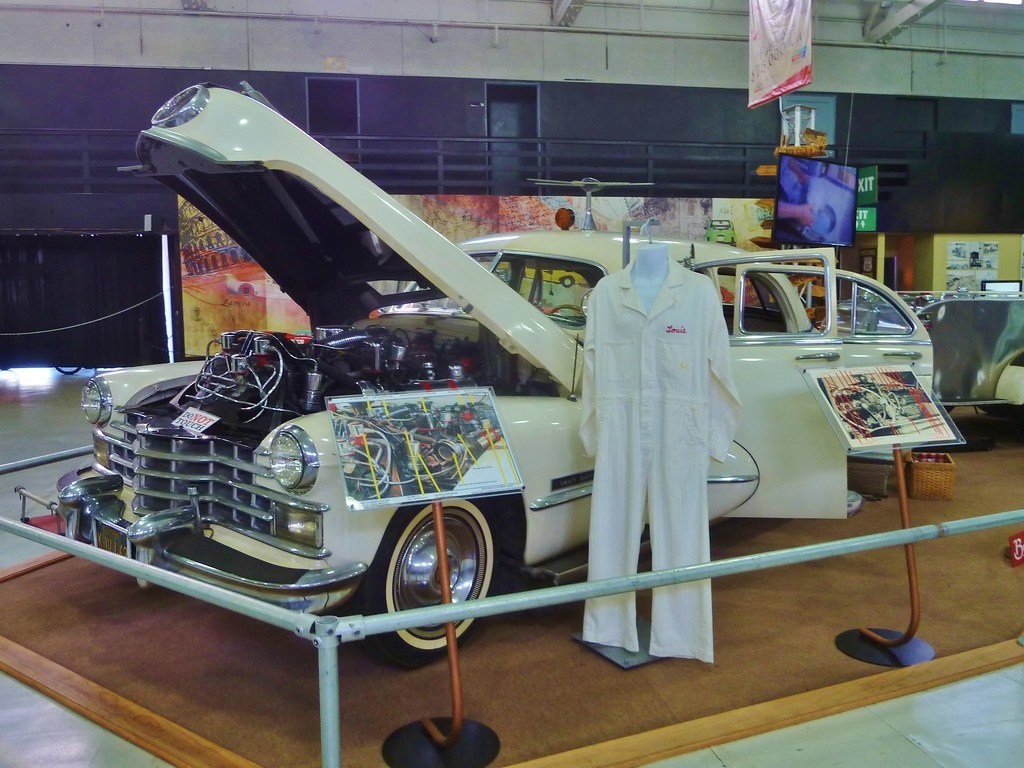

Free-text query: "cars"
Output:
<box><xmin>52</xmin><ymin>75</ymin><xmax>939</xmax><ymax>672</ymax></box>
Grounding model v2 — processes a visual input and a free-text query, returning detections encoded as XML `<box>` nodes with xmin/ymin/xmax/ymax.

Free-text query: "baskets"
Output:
<box><xmin>904</xmin><ymin>451</ymin><xmax>957</xmax><ymax>502</ymax></box>
